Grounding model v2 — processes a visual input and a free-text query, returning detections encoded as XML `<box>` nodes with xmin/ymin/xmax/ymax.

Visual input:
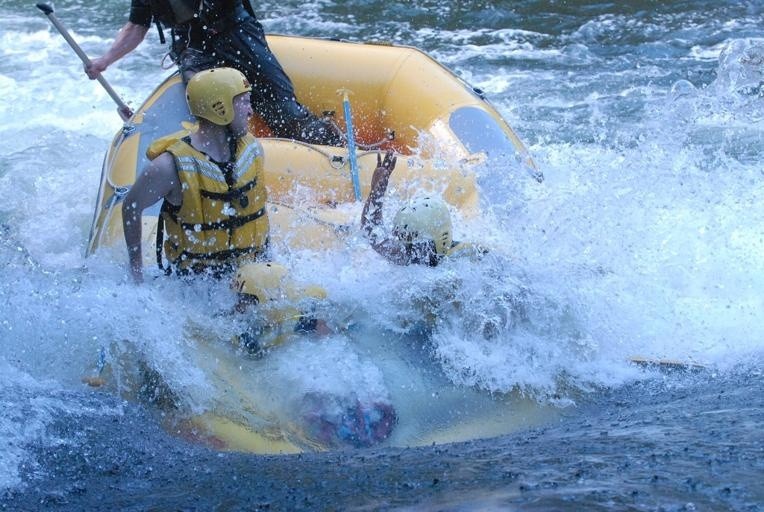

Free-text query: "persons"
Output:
<box><xmin>358</xmin><ymin>149</ymin><xmax>496</xmax><ymax>335</ymax></box>
<box><xmin>119</xmin><ymin>66</ymin><xmax>398</xmax><ymax>454</ymax></box>
<box><xmin>82</xmin><ymin>0</ymin><xmax>339</xmax><ymax>147</ymax></box>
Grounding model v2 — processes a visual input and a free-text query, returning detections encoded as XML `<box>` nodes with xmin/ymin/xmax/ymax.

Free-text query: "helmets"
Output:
<box><xmin>185</xmin><ymin>67</ymin><xmax>252</xmax><ymax>126</ymax></box>
<box><xmin>393</xmin><ymin>197</ymin><xmax>453</xmax><ymax>254</ymax></box>
<box><xmin>230</xmin><ymin>262</ymin><xmax>287</xmax><ymax>305</ymax></box>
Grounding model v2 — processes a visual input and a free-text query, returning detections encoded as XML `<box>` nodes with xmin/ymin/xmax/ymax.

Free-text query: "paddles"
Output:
<box><xmin>37</xmin><ymin>4</ymin><xmax>133</xmax><ymax>122</ymax></box>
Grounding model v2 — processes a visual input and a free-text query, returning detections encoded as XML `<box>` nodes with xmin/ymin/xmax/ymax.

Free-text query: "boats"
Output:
<box><xmin>84</xmin><ymin>35</ymin><xmax>581</xmax><ymax>454</ymax></box>
